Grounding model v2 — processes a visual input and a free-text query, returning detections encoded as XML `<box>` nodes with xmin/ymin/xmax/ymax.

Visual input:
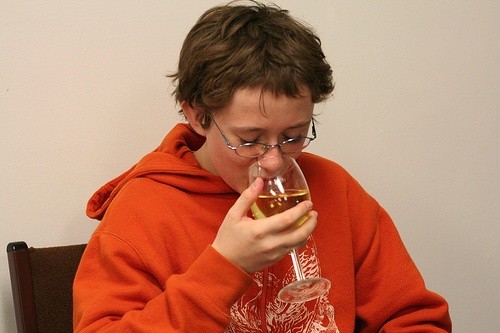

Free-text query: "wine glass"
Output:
<box><xmin>247</xmin><ymin>156</ymin><xmax>331</xmax><ymax>304</ymax></box>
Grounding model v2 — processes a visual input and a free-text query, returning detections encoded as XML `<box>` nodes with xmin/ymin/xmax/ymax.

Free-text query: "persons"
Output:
<box><xmin>72</xmin><ymin>6</ymin><xmax>459</xmax><ymax>333</ymax></box>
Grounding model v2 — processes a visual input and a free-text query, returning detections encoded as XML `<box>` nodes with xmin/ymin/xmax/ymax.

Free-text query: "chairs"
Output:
<box><xmin>7</xmin><ymin>242</ymin><xmax>87</xmax><ymax>333</ymax></box>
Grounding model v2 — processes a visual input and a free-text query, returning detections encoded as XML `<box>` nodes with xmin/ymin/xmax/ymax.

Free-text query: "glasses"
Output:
<box><xmin>207</xmin><ymin>113</ymin><xmax>318</xmax><ymax>159</ymax></box>
